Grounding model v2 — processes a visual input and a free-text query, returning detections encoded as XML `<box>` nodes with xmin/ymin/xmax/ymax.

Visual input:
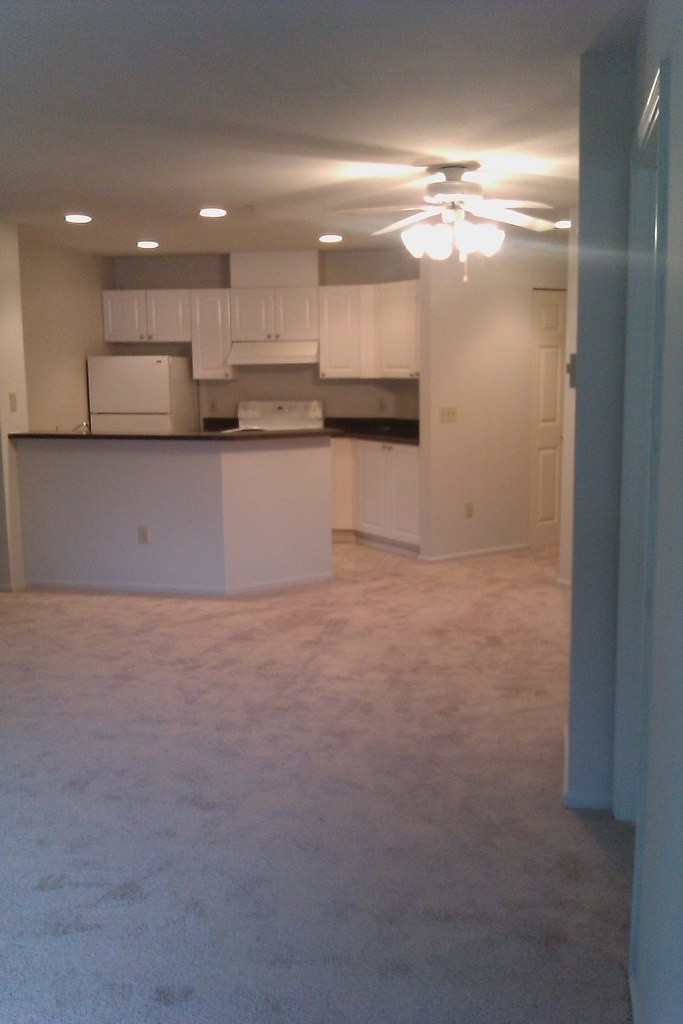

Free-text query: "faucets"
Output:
<box><xmin>72</xmin><ymin>422</ymin><xmax>87</xmax><ymax>432</ymax></box>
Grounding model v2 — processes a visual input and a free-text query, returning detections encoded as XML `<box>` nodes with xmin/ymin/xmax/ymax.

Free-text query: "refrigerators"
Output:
<box><xmin>85</xmin><ymin>353</ymin><xmax>200</xmax><ymax>435</ymax></box>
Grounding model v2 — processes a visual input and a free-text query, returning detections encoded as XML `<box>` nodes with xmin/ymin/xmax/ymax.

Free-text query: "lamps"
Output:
<box><xmin>399</xmin><ymin>207</ymin><xmax>506</xmax><ymax>281</ymax></box>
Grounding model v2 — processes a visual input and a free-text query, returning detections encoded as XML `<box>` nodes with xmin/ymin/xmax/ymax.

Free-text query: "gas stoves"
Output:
<box><xmin>221</xmin><ymin>398</ymin><xmax>325</xmax><ymax>433</ymax></box>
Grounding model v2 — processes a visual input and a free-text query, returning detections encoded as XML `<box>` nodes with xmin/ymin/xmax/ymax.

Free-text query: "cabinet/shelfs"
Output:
<box><xmin>320</xmin><ymin>284</ymin><xmax>361</xmax><ymax>379</ymax></box>
<box><xmin>101</xmin><ymin>288</ymin><xmax>192</xmax><ymax>343</ymax></box>
<box><xmin>191</xmin><ymin>289</ymin><xmax>241</xmax><ymax>380</ymax></box>
<box><xmin>354</xmin><ymin>438</ymin><xmax>419</xmax><ymax>544</ymax></box>
<box><xmin>331</xmin><ymin>438</ymin><xmax>354</xmax><ymax>529</ymax></box>
<box><xmin>374</xmin><ymin>281</ymin><xmax>419</xmax><ymax>381</ymax></box>
<box><xmin>231</xmin><ymin>286</ymin><xmax>318</xmax><ymax>341</ymax></box>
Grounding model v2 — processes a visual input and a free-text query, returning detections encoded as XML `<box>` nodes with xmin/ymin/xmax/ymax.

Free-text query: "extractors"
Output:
<box><xmin>226</xmin><ymin>340</ymin><xmax>320</xmax><ymax>366</ymax></box>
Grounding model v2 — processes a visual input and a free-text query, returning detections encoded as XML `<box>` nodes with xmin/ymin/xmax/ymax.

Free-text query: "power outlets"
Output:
<box><xmin>138</xmin><ymin>525</ymin><xmax>152</xmax><ymax>544</ymax></box>
<box><xmin>465</xmin><ymin>501</ymin><xmax>475</xmax><ymax>517</ymax></box>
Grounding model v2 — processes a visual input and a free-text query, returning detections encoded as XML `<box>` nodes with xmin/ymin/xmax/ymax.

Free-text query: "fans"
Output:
<box><xmin>337</xmin><ymin>166</ymin><xmax>556</xmax><ymax>237</ymax></box>
